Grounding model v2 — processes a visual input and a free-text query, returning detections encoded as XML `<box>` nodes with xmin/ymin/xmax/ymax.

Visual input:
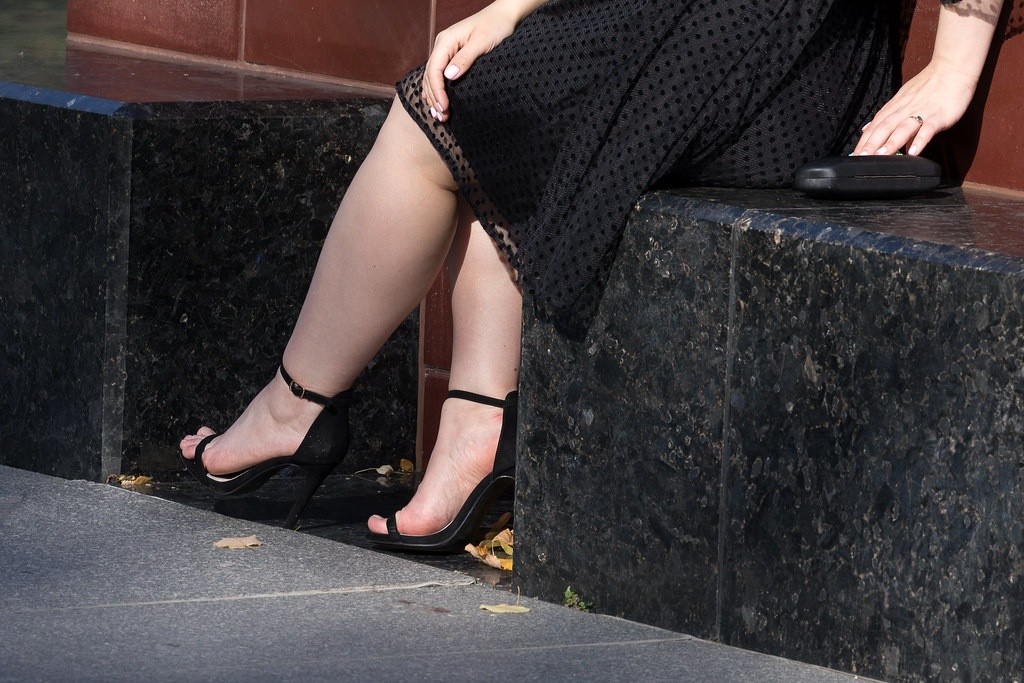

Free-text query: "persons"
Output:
<box><xmin>174</xmin><ymin>0</ymin><xmax>1024</xmax><ymax>555</ymax></box>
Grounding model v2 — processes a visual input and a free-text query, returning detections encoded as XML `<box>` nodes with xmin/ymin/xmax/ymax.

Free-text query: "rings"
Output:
<box><xmin>911</xmin><ymin>115</ymin><xmax>923</xmax><ymax>126</ymax></box>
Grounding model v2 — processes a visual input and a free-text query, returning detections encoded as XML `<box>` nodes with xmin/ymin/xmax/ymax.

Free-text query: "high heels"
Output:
<box><xmin>179</xmin><ymin>363</ymin><xmax>352</xmax><ymax>529</ymax></box>
<box><xmin>366</xmin><ymin>390</ymin><xmax>520</xmax><ymax>547</ymax></box>
